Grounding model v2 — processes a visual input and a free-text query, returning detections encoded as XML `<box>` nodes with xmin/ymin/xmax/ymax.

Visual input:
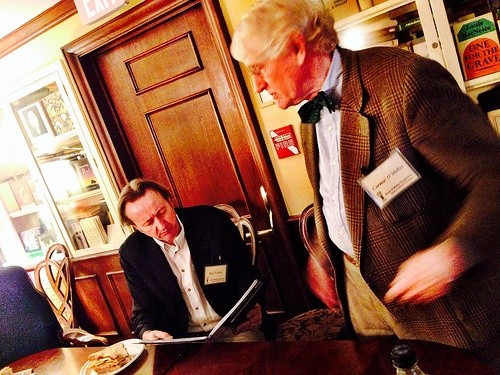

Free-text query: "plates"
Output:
<box><xmin>80</xmin><ymin>339</ymin><xmax>145</xmax><ymax>375</ymax></box>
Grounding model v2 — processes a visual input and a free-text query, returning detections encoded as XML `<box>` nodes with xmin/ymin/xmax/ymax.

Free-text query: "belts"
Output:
<box><xmin>344</xmin><ymin>252</ymin><xmax>357</xmax><ymax>266</ymax></box>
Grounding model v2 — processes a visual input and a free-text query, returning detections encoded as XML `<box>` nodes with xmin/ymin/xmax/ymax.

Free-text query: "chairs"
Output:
<box><xmin>299</xmin><ymin>204</ymin><xmax>335</xmax><ymax>280</ymax></box>
<box><xmin>0</xmin><ymin>244</ymin><xmax>78</xmax><ymax>367</ymax></box>
<box><xmin>214</xmin><ymin>204</ymin><xmax>257</xmax><ymax>268</ymax></box>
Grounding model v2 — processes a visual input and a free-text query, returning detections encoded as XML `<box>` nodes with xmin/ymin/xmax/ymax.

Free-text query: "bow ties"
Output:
<box><xmin>298</xmin><ymin>90</ymin><xmax>335</xmax><ymax>123</ymax></box>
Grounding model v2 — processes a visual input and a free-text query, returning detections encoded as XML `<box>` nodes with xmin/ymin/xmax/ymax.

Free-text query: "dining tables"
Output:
<box><xmin>0</xmin><ymin>339</ymin><xmax>498</xmax><ymax>375</ymax></box>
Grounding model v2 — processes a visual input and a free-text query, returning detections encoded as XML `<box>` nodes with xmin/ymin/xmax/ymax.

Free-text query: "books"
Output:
<box><xmin>134</xmin><ymin>279</ymin><xmax>262</xmax><ymax>344</ymax></box>
<box><xmin>452</xmin><ymin>11</ymin><xmax>500</xmax><ymax>81</ymax></box>
<box><xmin>327</xmin><ymin>0</ymin><xmax>428</xmax><ymax>58</ymax></box>
<box><xmin>0</xmin><ymin>146</ymin><xmax>115</xmax><ymax>250</ymax></box>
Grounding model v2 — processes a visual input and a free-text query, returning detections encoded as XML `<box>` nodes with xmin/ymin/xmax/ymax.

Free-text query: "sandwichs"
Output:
<box><xmin>87</xmin><ymin>343</ymin><xmax>132</xmax><ymax>375</ymax></box>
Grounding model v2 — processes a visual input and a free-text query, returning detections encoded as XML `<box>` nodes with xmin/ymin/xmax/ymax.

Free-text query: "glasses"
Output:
<box><xmin>245</xmin><ymin>63</ymin><xmax>263</xmax><ymax>75</ymax></box>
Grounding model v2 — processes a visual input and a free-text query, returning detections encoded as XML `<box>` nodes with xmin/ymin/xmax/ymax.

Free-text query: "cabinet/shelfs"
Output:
<box><xmin>0</xmin><ymin>60</ymin><xmax>134</xmax><ymax>269</ymax></box>
<box><xmin>313</xmin><ymin>0</ymin><xmax>500</xmax><ymax>130</ymax></box>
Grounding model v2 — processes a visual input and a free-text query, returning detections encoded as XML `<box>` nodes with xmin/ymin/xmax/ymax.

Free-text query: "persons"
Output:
<box><xmin>118</xmin><ymin>177</ymin><xmax>267</xmax><ymax>343</ymax></box>
<box><xmin>229</xmin><ymin>0</ymin><xmax>500</xmax><ymax>375</ymax></box>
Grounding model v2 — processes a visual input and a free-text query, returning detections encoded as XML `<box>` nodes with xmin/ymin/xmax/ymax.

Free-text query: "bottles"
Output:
<box><xmin>390</xmin><ymin>344</ymin><xmax>424</xmax><ymax>375</ymax></box>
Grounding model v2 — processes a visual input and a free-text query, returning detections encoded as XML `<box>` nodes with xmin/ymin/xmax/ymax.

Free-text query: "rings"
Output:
<box><xmin>251</xmin><ymin>324</ymin><xmax>256</xmax><ymax>328</ymax></box>
